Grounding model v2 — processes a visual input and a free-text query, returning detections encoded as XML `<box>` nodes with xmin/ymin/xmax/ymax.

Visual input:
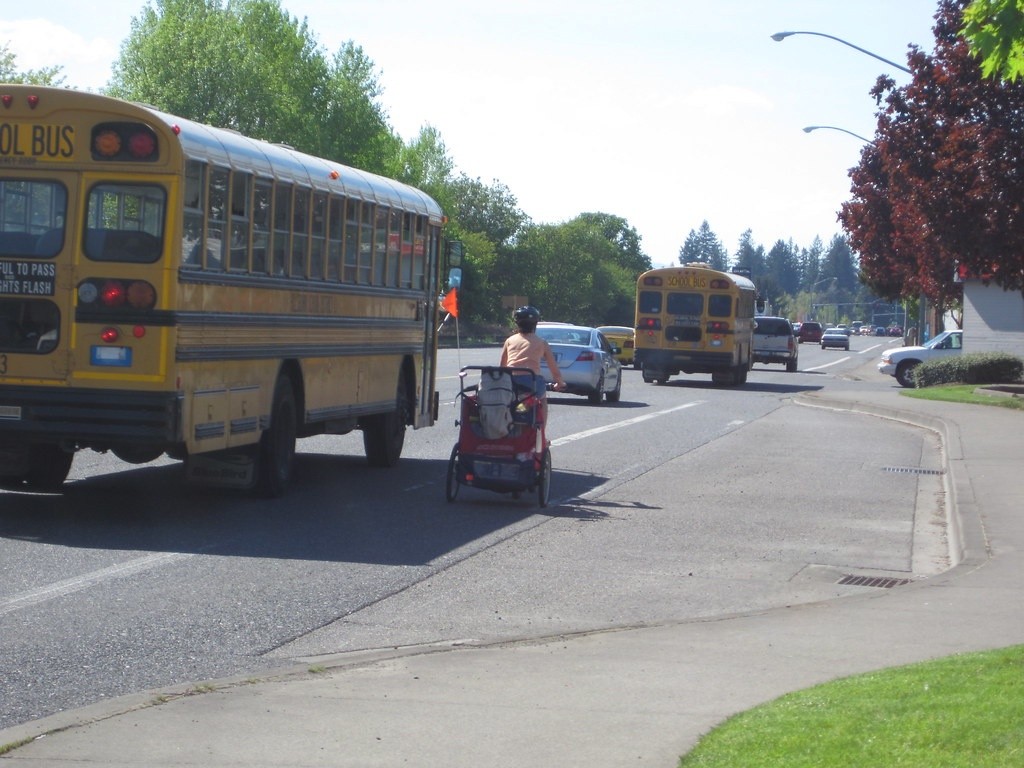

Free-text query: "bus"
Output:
<box><xmin>635</xmin><ymin>261</ymin><xmax>766</xmax><ymax>386</ymax></box>
<box><xmin>1</xmin><ymin>83</ymin><xmax>467</xmax><ymax>497</ymax></box>
<box><xmin>851</xmin><ymin>321</ymin><xmax>862</xmax><ymax>334</ymax></box>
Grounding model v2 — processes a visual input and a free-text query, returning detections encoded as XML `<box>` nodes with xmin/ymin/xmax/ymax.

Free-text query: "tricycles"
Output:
<box><xmin>445</xmin><ymin>362</ymin><xmax>565</xmax><ymax>505</ymax></box>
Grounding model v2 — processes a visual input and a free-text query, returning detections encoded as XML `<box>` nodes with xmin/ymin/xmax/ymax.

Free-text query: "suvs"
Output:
<box><xmin>799</xmin><ymin>322</ymin><xmax>822</xmax><ymax>344</ymax></box>
<box><xmin>750</xmin><ymin>315</ymin><xmax>799</xmax><ymax>372</ymax></box>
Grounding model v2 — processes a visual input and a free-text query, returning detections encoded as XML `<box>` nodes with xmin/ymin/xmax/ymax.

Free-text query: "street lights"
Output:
<box><xmin>810</xmin><ymin>276</ymin><xmax>838</xmax><ymax>320</ymax></box>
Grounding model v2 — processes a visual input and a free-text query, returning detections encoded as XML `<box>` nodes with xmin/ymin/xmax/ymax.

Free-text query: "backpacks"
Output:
<box><xmin>476</xmin><ymin>368</ymin><xmax>516</xmax><ymax>440</ymax></box>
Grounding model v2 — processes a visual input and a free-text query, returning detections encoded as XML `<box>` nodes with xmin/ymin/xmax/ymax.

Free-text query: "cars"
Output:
<box><xmin>792</xmin><ymin>322</ymin><xmax>802</xmax><ymax>336</ymax></box>
<box><xmin>850</xmin><ymin>325</ymin><xmax>904</xmax><ymax>336</ymax></box>
<box><xmin>822</xmin><ymin>327</ymin><xmax>850</xmax><ymax>351</ymax></box>
<box><xmin>877</xmin><ymin>328</ymin><xmax>962</xmax><ymax>387</ymax></box>
<box><xmin>534</xmin><ymin>321</ymin><xmax>624</xmax><ymax>402</ymax></box>
<box><xmin>596</xmin><ymin>327</ymin><xmax>635</xmax><ymax>368</ymax></box>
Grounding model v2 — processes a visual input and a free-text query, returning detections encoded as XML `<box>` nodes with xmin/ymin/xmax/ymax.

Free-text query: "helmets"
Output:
<box><xmin>514</xmin><ymin>305</ymin><xmax>540</xmax><ymax>324</ymax></box>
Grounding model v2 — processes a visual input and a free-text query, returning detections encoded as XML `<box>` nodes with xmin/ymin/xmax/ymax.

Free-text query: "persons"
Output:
<box><xmin>500</xmin><ymin>306</ymin><xmax>566</xmax><ymax>445</ymax></box>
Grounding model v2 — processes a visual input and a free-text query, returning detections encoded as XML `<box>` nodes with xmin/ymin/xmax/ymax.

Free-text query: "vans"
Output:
<box><xmin>837</xmin><ymin>324</ymin><xmax>847</xmax><ymax>329</ymax></box>
